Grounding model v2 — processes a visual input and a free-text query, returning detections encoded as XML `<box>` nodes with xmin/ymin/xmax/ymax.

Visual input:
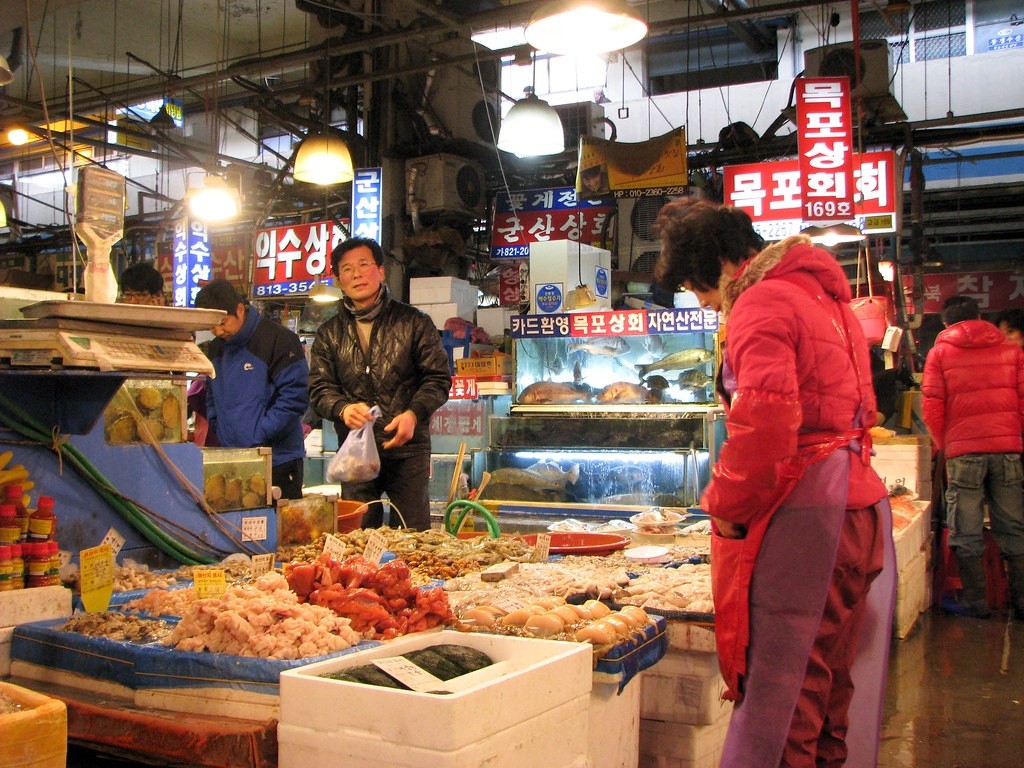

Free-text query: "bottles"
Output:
<box><xmin>26</xmin><ymin>496</ymin><xmax>57</xmax><ymax>541</ymax></box>
<box><xmin>0</xmin><ymin>504</ymin><xmax>20</xmax><ymax>538</ymax></box>
<box><xmin>0</xmin><ymin>528</ymin><xmax>60</xmax><ymax>590</ymax></box>
<box><xmin>504</xmin><ymin>329</ymin><xmax>510</xmax><ymax>354</ymax></box>
<box><xmin>6</xmin><ymin>485</ymin><xmax>27</xmax><ymax>536</ymax></box>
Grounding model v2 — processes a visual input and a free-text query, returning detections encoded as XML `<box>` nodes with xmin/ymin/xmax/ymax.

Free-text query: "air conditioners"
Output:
<box><xmin>308</xmin><ymin>13</ymin><xmax>363</xmax><ymax>98</ymax></box>
<box><xmin>405</xmin><ymin>154</ymin><xmax>486</xmax><ymax>218</ymax></box>
<box><xmin>804</xmin><ymin>39</ymin><xmax>895</xmax><ymax>99</ymax></box>
<box><xmin>425</xmin><ymin>37</ymin><xmax>501</xmax><ymax>151</ymax></box>
<box><xmin>550</xmin><ymin>101</ymin><xmax>605</xmax><ymax>154</ymax></box>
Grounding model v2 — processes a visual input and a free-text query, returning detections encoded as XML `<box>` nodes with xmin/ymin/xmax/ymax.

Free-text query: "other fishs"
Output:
<box><xmin>516</xmin><ymin>334</ymin><xmax>717</xmax><ymax>406</ymax></box>
<box><xmin>289</xmin><ymin>455</ymin><xmax>717</xmax><ymax>645</ymax></box>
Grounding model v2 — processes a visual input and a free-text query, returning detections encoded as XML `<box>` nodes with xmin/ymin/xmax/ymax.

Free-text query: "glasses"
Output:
<box><xmin>122</xmin><ymin>291</ymin><xmax>150</xmax><ymax>300</ymax></box>
<box><xmin>339</xmin><ymin>259</ymin><xmax>376</xmax><ymax>279</ymax></box>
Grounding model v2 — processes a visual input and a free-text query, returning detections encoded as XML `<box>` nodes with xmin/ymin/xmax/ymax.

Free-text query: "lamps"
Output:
<box><xmin>294</xmin><ymin>0</ymin><xmax>354</xmax><ymax>185</ymax></box>
<box><xmin>800</xmin><ymin>224</ymin><xmax>866</xmax><ymax>246</ymax></box>
<box><xmin>181</xmin><ymin>169</ymin><xmax>246</xmax><ymax>227</ymax></box>
<box><xmin>0</xmin><ymin>54</ymin><xmax>15</xmax><ymax>86</ymax></box>
<box><xmin>150</xmin><ymin>91</ymin><xmax>176</xmax><ymax>129</ymax></box>
<box><xmin>525</xmin><ymin>0</ymin><xmax>647</xmax><ymax>54</ymax></box>
<box><xmin>496</xmin><ymin>51</ymin><xmax>565</xmax><ymax>156</ymax></box>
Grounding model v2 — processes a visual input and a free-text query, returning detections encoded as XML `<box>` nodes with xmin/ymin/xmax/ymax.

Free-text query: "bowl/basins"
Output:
<box><xmin>338</xmin><ymin>501</ymin><xmax>368</xmax><ymax>532</ymax></box>
<box><xmin>624</xmin><ymin>546</ymin><xmax>668</xmax><ymax>565</ymax></box>
<box><xmin>513</xmin><ymin>532</ymin><xmax>630</xmax><ymax>556</ymax></box>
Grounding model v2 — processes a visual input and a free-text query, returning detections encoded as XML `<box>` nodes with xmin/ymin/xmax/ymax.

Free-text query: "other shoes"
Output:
<box><xmin>955</xmin><ymin>598</ymin><xmax>991</xmax><ymax>618</ymax></box>
<box><xmin>1008</xmin><ymin>607</ymin><xmax>1024</xmax><ymax>624</ymax></box>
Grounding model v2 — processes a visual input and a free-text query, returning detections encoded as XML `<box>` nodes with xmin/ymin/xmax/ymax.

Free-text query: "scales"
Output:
<box><xmin>0</xmin><ymin>298</ymin><xmax>233</xmax><ymax>380</ymax></box>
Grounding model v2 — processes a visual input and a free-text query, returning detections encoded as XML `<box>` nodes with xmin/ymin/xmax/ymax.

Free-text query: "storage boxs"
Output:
<box><xmin>105</xmin><ymin>378</ymin><xmax>187</xmax><ymax>445</ymax></box>
<box><xmin>304</xmin><ymin>240</ymin><xmax>725</xmax><ymax>511</ymax></box>
<box><xmin>870</xmin><ymin>434</ymin><xmax>932</xmax><ymax>638</ymax></box>
<box><xmin>201</xmin><ymin>447</ymin><xmax>272</xmax><ymax>512</ymax></box>
<box><xmin>276</xmin><ymin>495</ymin><xmax>339</xmax><ymax>548</ymax></box>
<box><xmin>0</xmin><ymin>562</ymin><xmax>734</xmax><ymax>768</ymax></box>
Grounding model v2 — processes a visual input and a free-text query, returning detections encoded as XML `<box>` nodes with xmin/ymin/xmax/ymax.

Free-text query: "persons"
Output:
<box><xmin>119</xmin><ymin>265</ymin><xmax>320</xmax><ymax>501</ymax></box>
<box><xmin>306</xmin><ymin>237</ymin><xmax>453</xmax><ymax>531</ymax></box>
<box><xmin>654</xmin><ymin>201</ymin><xmax>897</xmax><ymax>768</ymax></box>
<box><xmin>872</xmin><ymin>295</ymin><xmax>1024</xmax><ymax>618</ymax></box>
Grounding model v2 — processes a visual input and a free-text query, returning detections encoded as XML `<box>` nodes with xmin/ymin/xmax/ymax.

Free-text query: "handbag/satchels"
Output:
<box><xmin>325</xmin><ymin>404</ymin><xmax>382</xmax><ymax>483</ymax></box>
